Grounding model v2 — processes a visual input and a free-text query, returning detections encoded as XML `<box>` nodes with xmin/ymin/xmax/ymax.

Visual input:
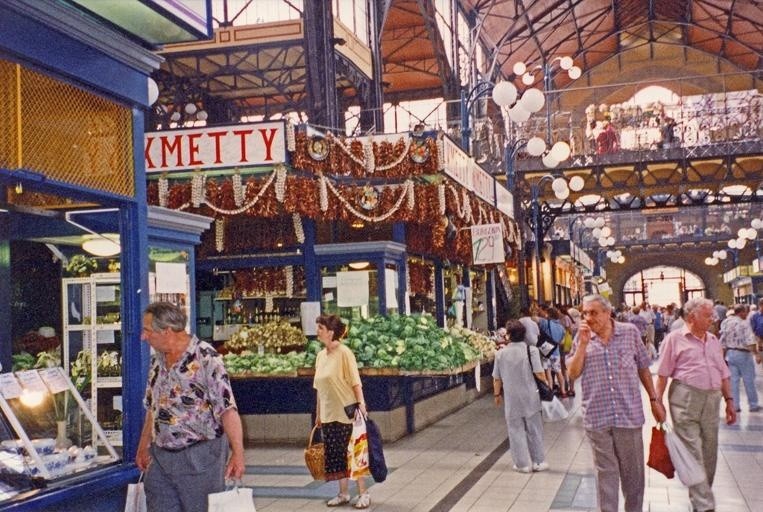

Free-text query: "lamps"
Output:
<box><xmin>506</xmin><ymin>138</ymin><xmax>571</xmax><ymax>183</ymax></box>
<box><xmin>79</xmin><ymin>233</ymin><xmax>121</xmax><ymax>257</ymax></box>
<box><xmin>534</xmin><ymin>173</ymin><xmax>585</xmax><ymax>201</ymax></box>
<box><xmin>170</xmin><ymin>103</ymin><xmax>208</xmax><ymax>128</ymax></box>
<box><xmin>705</xmin><ymin>218</ymin><xmax>763</xmax><ymax>266</ymax></box>
<box><xmin>465</xmin><ymin>79</ymin><xmax>546</xmax><ymax>142</ymax></box>
<box><xmin>147</xmin><ymin>78</ymin><xmax>159</xmax><ymax>106</ymax></box>
<box><xmin>491</xmin><ymin>79</ymin><xmax>546</xmax><ymax>123</ymax></box>
<box><xmin>578</xmin><ymin>192</ymin><xmax>601</xmax><ymax>206</ymax></box>
<box><xmin>512</xmin><ymin>55</ymin><xmax>583</xmax><ymax>85</ymax></box>
<box><xmin>569</xmin><ymin>216</ymin><xmax>604</xmax><ymax>240</ymax></box>
<box><xmin>508</xmin><ymin>136</ymin><xmax>571</xmax><ymax>168</ymax></box>
<box><xmin>593</xmin><ymin>226</ymin><xmax>625</xmax><ymax>268</ymax></box>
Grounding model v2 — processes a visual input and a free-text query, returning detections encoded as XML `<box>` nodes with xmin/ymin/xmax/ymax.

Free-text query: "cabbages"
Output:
<box><xmin>340</xmin><ymin>313</ymin><xmax>479</xmax><ymax>370</ymax></box>
<box><xmin>222</xmin><ymin>339</ymin><xmax>325</xmax><ymax>374</ymax></box>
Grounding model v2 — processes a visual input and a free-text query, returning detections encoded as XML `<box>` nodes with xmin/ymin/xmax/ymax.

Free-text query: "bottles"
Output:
<box><xmin>254</xmin><ymin>307</ymin><xmax>281</xmax><ymax>323</ymax></box>
<box><xmin>227</xmin><ymin>308</ymin><xmax>252</xmax><ymax>324</ymax></box>
<box><xmin>284</xmin><ymin>307</ymin><xmax>300</xmax><ymax>320</ymax></box>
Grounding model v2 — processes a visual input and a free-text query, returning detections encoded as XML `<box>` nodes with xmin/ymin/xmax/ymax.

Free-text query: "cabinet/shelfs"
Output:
<box><xmin>61</xmin><ymin>273</ymin><xmax>193</xmax><ymax>456</ymax></box>
<box><xmin>213</xmin><ymin>296</ymin><xmax>309</xmax><ymax>347</ymax></box>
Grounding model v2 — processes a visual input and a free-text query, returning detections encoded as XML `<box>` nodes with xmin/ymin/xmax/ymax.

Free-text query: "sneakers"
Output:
<box><xmin>568</xmin><ymin>390</ymin><xmax>576</xmax><ymax>397</ymax></box>
<box><xmin>533</xmin><ymin>462</ymin><xmax>550</xmax><ymax>472</ymax></box>
<box><xmin>512</xmin><ymin>463</ymin><xmax>532</xmax><ymax>473</ymax></box>
<box><xmin>561</xmin><ymin>392</ymin><xmax>568</xmax><ymax>398</ymax></box>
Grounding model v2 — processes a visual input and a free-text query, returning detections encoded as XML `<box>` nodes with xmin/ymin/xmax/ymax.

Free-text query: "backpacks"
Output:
<box><xmin>536</xmin><ymin>328</ymin><xmax>558</xmax><ymax>359</ymax></box>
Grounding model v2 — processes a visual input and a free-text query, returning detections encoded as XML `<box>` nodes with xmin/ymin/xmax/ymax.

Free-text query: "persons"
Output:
<box><xmin>652</xmin><ymin>112</ymin><xmax>680</xmax><ymax>150</ymax></box>
<box><xmin>596</xmin><ymin>122</ymin><xmax>620</xmax><ymax>153</ymax></box>
<box><xmin>556</xmin><ymin>305</ymin><xmax>577</xmax><ymax>398</ymax></box>
<box><xmin>564</xmin><ymin>294</ymin><xmax>665</xmax><ymax>512</ymax></box>
<box><xmin>714</xmin><ymin>298</ymin><xmax>762</xmax><ymax>413</ymax></box>
<box><xmin>536</xmin><ymin>304</ymin><xmax>548</xmax><ymax>315</ymax></box>
<box><xmin>134</xmin><ymin>301</ymin><xmax>245</xmax><ymax>512</ymax></box>
<box><xmin>567</xmin><ymin>302</ymin><xmax>580</xmax><ymax>334</ymax></box>
<box><xmin>313</xmin><ymin>314</ymin><xmax>371</xmax><ymax>509</ymax></box>
<box><xmin>519</xmin><ymin>308</ymin><xmax>540</xmax><ymax>345</ymax></box>
<box><xmin>586</xmin><ymin>120</ymin><xmax>596</xmax><ymax>154</ymax></box>
<box><xmin>610</xmin><ymin>299</ymin><xmax>687</xmax><ymax>357</ymax></box>
<box><xmin>533</xmin><ymin>308</ymin><xmax>568</xmax><ymax>400</ymax></box>
<box><xmin>493</xmin><ymin>319</ymin><xmax>549</xmax><ymax>473</ymax></box>
<box><xmin>656</xmin><ymin>296</ymin><xmax>736</xmax><ymax>512</ymax></box>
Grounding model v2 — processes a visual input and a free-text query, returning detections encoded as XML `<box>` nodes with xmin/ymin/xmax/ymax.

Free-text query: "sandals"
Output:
<box><xmin>354</xmin><ymin>493</ymin><xmax>372</xmax><ymax>510</ymax></box>
<box><xmin>325</xmin><ymin>491</ymin><xmax>351</xmax><ymax>507</ymax></box>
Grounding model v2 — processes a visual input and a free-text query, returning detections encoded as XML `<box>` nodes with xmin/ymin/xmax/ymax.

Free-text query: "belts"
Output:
<box><xmin>726</xmin><ymin>348</ymin><xmax>751</xmax><ymax>353</ymax></box>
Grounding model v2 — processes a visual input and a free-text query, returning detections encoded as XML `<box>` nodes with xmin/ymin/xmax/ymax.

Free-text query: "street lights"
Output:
<box><xmin>528</xmin><ymin>173</ymin><xmax>586</xmax><ymax>325</ymax></box>
<box><xmin>503</xmin><ymin>133</ymin><xmax>570</xmax><ymax>333</ymax></box>
<box><xmin>704</xmin><ymin>218</ymin><xmax>762</xmax><ymax>303</ymax></box>
<box><xmin>458</xmin><ymin>79</ymin><xmax>546</xmax><ymax>388</ymax></box>
<box><xmin>570</xmin><ymin>209</ymin><xmax>624</xmax><ymax>330</ymax></box>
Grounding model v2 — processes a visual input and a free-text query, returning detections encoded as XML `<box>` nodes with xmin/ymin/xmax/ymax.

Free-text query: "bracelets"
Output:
<box><xmin>492</xmin><ymin>393</ymin><xmax>502</xmax><ymax>400</ymax></box>
<box><xmin>724</xmin><ymin>396</ymin><xmax>735</xmax><ymax>401</ymax></box>
<box><xmin>650</xmin><ymin>396</ymin><xmax>660</xmax><ymax>404</ymax></box>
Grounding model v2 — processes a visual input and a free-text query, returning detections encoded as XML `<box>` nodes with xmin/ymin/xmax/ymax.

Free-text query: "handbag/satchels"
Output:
<box><xmin>122</xmin><ymin>470</ymin><xmax>148</xmax><ymax>512</ymax></box>
<box><xmin>362</xmin><ymin>414</ymin><xmax>387</xmax><ymax>483</ymax></box>
<box><xmin>524</xmin><ymin>372</ymin><xmax>553</xmax><ymax>402</ymax></box>
<box><xmin>647</xmin><ymin>420</ymin><xmax>676</xmax><ymax>481</ymax></box>
<box><xmin>664</xmin><ymin>421</ymin><xmax>706</xmax><ymax>488</ymax></box>
<box><xmin>346</xmin><ymin>409</ymin><xmax>370</xmax><ymax>482</ymax></box>
<box><xmin>559</xmin><ymin>328</ymin><xmax>573</xmax><ymax>355</ymax></box>
<box><xmin>304</xmin><ymin>421</ymin><xmax>330</xmax><ymax>483</ymax></box>
<box><xmin>204</xmin><ymin>479</ymin><xmax>258</xmax><ymax>512</ymax></box>
